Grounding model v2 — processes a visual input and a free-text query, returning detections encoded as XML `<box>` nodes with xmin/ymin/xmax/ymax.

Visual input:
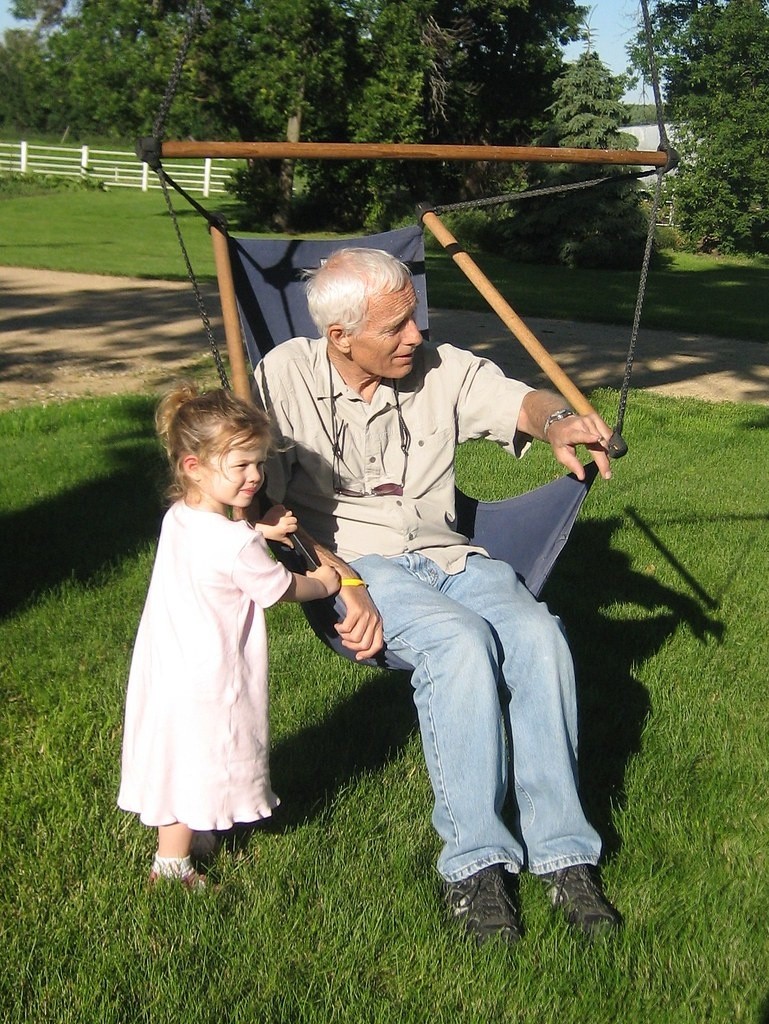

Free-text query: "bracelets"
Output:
<box><xmin>341</xmin><ymin>579</ymin><xmax>368</xmax><ymax>588</ymax></box>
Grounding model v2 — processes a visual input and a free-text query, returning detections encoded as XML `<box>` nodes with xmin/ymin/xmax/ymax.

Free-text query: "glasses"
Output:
<box><xmin>332</xmin><ymin>414</ymin><xmax>408</xmax><ymax>497</ymax></box>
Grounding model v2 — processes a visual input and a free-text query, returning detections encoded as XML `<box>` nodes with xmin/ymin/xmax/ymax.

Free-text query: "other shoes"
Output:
<box><xmin>192</xmin><ymin>840</ymin><xmax>250</xmax><ymax>866</ymax></box>
<box><xmin>150</xmin><ymin>867</ymin><xmax>221</xmax><ymax>893</ymax></box>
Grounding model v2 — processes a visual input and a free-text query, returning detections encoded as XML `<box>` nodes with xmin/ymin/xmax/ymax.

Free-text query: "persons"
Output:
<box><xmin>117</xmin><ymin>383</ymin><xmax>340</xmax><ymax>891</ymax></box>
<box><xmin>254</xmin><ymin>247</ymin><xmax>624</xmax><ymax>941</ymax></box>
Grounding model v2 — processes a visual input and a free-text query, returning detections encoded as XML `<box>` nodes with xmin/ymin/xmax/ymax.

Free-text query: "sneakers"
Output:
<box><xmin>444</xmin><ymin>863</ymin><xmax>521</xmax><ymax>947</ymax></box>
<box><xmin>540</xmin><ymin>863</ymin><xmax>618</xmax><ymax>934</ymax></box>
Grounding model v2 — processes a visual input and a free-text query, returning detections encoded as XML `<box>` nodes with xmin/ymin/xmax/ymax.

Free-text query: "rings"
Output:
<box><xmin>597</xmin><ymin>437</ymin><xmax>605</xmax><ymax>441</ymax></box>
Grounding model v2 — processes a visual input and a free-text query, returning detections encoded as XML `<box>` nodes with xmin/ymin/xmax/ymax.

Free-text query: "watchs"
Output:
<box><xmin>543</xmin><ymin>409</ymin><xmax>572</xmax><ymax>434</ymax></box>
